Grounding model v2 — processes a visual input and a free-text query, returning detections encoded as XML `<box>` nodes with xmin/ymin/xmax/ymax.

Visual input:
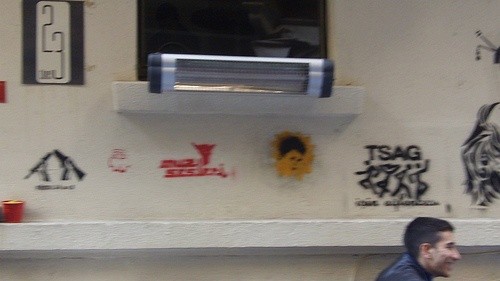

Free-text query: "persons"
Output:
<box><xmin>375</xmin><ymin>214</ymin><xmax>461</xmax><ymax>280</ymax></box>
